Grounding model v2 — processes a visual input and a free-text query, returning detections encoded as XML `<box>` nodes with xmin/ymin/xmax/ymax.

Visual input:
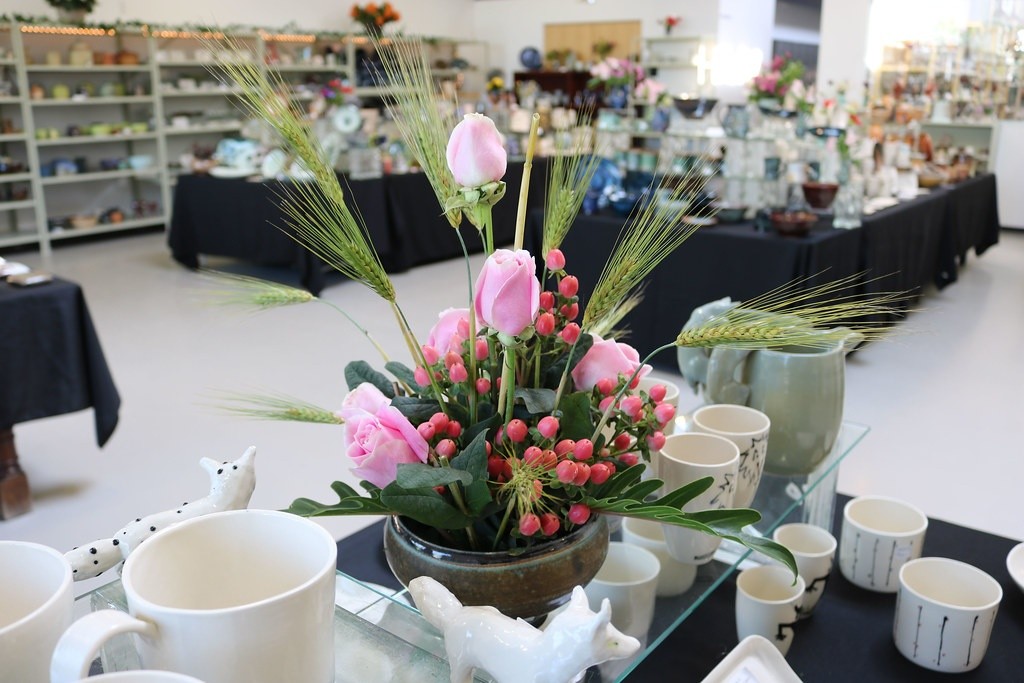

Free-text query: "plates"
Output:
<box><xmin>0</xmin><ymin>257</ymin><xmax>56</xmax><ymax>288</ymax></box>
<box><xmin>1006</xmin><ymin>541</ymin><xmax>1024</xmax><ymax>588</ymax></box>
<box><xmin>207</xmin><ymin>102</ymin><xmax>360</xmax><ymax>184</ymax></box>
<box><xmin>697</xmin><ymin>636</ymin><xmax>804</xmax><ymax>683</ymax></box>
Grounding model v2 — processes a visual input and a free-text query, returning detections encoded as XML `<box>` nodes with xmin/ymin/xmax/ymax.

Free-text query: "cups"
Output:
<box><xmin>835</xmin><ymin>496</ymin><xmax>929</xmax><ymax>593</ymax></box>
<box><xmin>0</xmin><ymin>40</ymin><xmax>161</xmax><ymax>237</ymax></box>
<box><xmin>585</xmin><ymin>539</ymin><xmax>661</xmax><ymax>638</ymax></box>
<box><xmin>692</xmin><ymin>402</ymin><xmax>771</xmax><ymax>508</ymax></box>
<box><xmin>631</xmin><ymin>376</ymin><xmax>679</xmax><ymax>497</ymax></box>
<box><xmin>264</xmin><ymin>40</ymin><xmax>348</xmax><ymax>113</ymax></box>
<box><xmin>893</xmin><ymin>556</ymin><xmax>1003</xmax><ymax>674</ymax></box>
<box><xmin>773</xmin><ymin>523</ymin><xmax>836</xmax><ymax>613</ymax></box>
<box><xmin>602</xmin><ymin>365</ymin><xmax>655</xmax><ymax>447</ymax></box>
<box><xmin>737</xmin><ymin>567</ymin><xmax>806</xmax><ymax>659</ymax></box>
<box><xmin>659</xmin><ymin>431</ymin><xmax>739</xmax><ymax>566</ymax></box>
<box><xmin>155</xmin><ymin>37</ymin><xmax>256</xmax><ymax>190</ymax></box>
<box><xmin>50</xmin><ymin>508</ymin><xmax>338</xmax><ymax>683</ymax></box>
<box><xmin>69</xmin><ymin>669</ymin><xmax>206</xmax><ymax>683</ymax></box>
<box><xmin>621</xmin><ymin>515</ymin><xmax>698</xmax><ymax>598</ymax></box>
<box><xmin>1</xmin><ymin>540</ymin><xmax>73</xmax><ymax>683</ymax></box>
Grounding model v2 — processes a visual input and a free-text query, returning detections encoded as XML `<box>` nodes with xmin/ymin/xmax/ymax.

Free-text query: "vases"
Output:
<box><xmin>366</xmin><ymin>22</ymin><xmax>382</xmax><ymax>36</ymax></box>
<box><xmin>383</xmin><ymin>514</ymin><xmax>611</xmax><ymax>619</ymax></box>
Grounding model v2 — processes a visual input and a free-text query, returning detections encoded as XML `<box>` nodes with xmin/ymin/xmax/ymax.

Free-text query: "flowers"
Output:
<box><xmin>193</xmin><ymin>22</ymin><xmax>924</xmax><ymax>586</ymax></box>
<box><xmin>350</xmin><ymin>2</ymin><xmax>399</xmax><ymax>28</ymax></box>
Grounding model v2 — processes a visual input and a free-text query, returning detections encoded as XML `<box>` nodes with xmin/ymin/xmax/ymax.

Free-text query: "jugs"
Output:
<box><xmin>673</xmin><ymin>297</ymin><xmax>865</xmax><ymax>479</ymax></box>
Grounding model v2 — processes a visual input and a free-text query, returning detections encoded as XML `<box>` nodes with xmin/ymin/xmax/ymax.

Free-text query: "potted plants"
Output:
<box><xmin>48</xmin><ymin>0</ymin><xmax>98</xmax><ymax>24</ymax></box>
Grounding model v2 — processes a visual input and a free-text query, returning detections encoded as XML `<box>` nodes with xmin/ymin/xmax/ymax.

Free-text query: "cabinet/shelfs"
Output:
<box><xmin>0</xmin><ymin>18</ymin><xmax>489</xmax><ymax>259</ymax></box>
<box><xmin>641</xmin><ymin>36</ymin><xmax>709</xmax><ymax>69</ymax></box>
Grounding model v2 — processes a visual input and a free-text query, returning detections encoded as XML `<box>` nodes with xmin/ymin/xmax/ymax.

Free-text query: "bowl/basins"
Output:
<box><xmin>577</xmin><ymin>94</ymin><xmax>840</xmax><ymax>235</ymax></box>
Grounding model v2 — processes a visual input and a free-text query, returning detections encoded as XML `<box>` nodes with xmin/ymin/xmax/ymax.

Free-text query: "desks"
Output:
<box><xmin>0</xmin><ymin>274</ymin><xmax>121</xmax><ymax>520</ymax></box>
<box><xmin>526</xmin><ymin>173</ymin><xmax>1000</xmax><ymax>374</ymax></box>
<box><xmin>168</xmin><ymin>155</ymin><xmax>583</xmax><ymax>297</ymax></box>
<box><xmin>73</xmin><ymin>486</ymin><xmax>1024</xmax><ymax>682</ymax></box>
